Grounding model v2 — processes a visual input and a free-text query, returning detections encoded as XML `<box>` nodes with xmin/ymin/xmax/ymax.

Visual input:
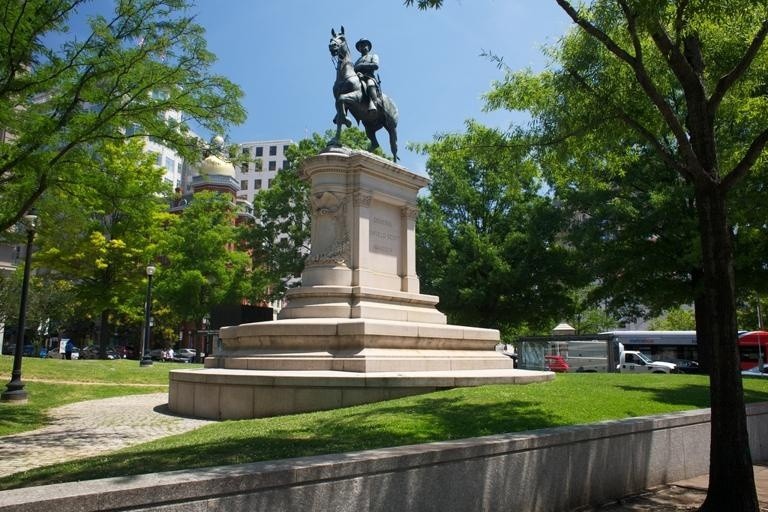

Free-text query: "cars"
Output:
<box><xmin>741</xmin><ymin>365</ymin><xmax>768</xmax><ymax>379</ymax></box>
<box><xmin>45</xmin><ymin>344</ymin><xmax>206</xmax><ymax>363</ymax></box>
<box><xmin>662</xmin><ymin>357</ymin><xmax>699</xmax><ymax>375</ymax></box>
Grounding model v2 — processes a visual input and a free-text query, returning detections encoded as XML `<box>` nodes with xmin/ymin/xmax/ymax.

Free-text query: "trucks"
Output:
<box><xmin>520</xmin><ymin>339</ymin><xmax>678</xmax><ymax>374</ymax></box>
<box><xmin>588</xmin><ymin>329</ymin><xmax>768</xmax><ymax>373</ymax></box>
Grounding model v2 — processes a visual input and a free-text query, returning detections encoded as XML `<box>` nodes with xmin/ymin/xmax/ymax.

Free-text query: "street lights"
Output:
<box><xmin>1</xmin><ymin>207</ymin><xmax>42</xmax><ymax>404</ymax></box>
<box><xmin>138</xmin><ymin>264</ymin><xmax>155</xmax><ymax>367</ymax></box>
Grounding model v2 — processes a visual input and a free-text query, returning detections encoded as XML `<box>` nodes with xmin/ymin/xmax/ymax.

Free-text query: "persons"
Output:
<box><xmin>333</xmin><ymin>38</ymin><xmax>382</xmax><ymax>125</ymax></box>
<box><xmin>160</xmin><ymin>347</ymin><xmax>167</xmax><ymax>362</ymax></box>
<box><xmin>166</xmin><ymin>345</ymin><xmax>175</xmax><ymax>362</ymax></box>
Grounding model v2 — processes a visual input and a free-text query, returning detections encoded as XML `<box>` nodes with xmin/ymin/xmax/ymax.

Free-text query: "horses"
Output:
<box><xmin>327</xmin><ymin>24</ymin><xmax>399</xmax><ymax>163</ymax></box>
